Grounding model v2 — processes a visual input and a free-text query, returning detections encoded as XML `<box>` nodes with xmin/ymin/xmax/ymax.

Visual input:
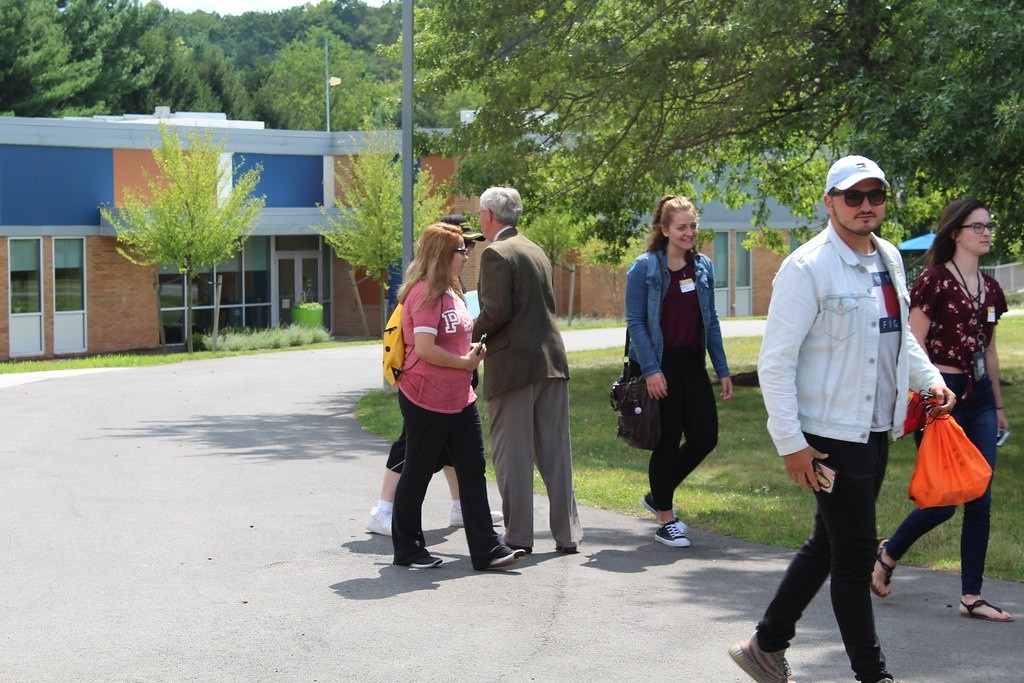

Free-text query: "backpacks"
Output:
<box><xmin>383</xmin><ymin>279</ymin><xmax>444</xmax><ymax>386</ymax></box>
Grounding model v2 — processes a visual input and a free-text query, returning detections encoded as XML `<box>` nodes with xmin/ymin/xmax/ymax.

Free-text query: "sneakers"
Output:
<box><xmin>489</xmin><ymin>545</ymin><xmax>526</xmax><ymax>568</ymax></box>
<box><xmin>366</xmin><ymin>508</ymin><xmax>392</xmax><ymax>536</ymax></box>
<box><xmin>641</xmin><ymin>493</ymin><xmax>689</xmax><ymax>533</ymax></box>
<box><xmin>410</xmin><ymin>555</ymin><xmax>442</xmax><ymax>569</ymax></box>
<box><xmin>655</xmin><ymin>521</ymin><xmax>691</xmax><ymax>547</ymax></box>
<box><xmin>450</xmin><ymin>505</ymin><xmax>503</xmax><ymax>526</ymax></box>
<box><xmin>729</xmin><ymin>631</ymin><xmax>795</xmax><ymax>683</ymax></box>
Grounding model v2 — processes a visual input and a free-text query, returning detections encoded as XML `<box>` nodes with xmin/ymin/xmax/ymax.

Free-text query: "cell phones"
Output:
<box><xmin>476</xmin><ymin>335</ymin><xmax>486</xmax><ymax>357</ymax></box>
<box><xmin>805</xmin><ymin>460</ymin><xmax>838</xmax><ymax>494</ymax></box>
<box><xmin>996</xmin><ymin>429</ymin><xmax>1010</xmax><ymax>447</ymax></box>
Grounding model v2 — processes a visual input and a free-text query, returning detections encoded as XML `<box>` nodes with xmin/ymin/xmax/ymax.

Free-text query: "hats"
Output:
<box><xmin>825</xmin><ymin>155</ymin><xmax>890</xmax><ymax>195</ymax></box>
<box><xmin>441</xmin><ymin>215</ymin><xmax>486</xmax><ymax>242</ymax></box>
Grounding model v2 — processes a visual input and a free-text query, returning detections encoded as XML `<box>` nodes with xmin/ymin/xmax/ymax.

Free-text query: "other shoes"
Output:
<box><xmin>506</xmin><ymin>544</ymin><xmax>533</xmax><ymax>554</ymax></box>
<box><xmin>556</xmin><ymin>542</ymin><xmax>577</xmax><ymax>554</ymax></box>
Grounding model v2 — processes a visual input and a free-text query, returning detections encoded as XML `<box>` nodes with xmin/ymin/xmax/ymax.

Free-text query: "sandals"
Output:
<box><xmin>870</xmin><ymin>539</ymin><xmax>896</xmax><ymax>597</ymax></box>
<box><xmin>960</xmin><ymin>600</ymin><xmax>1015</xmax><ymax>622</ymax></box>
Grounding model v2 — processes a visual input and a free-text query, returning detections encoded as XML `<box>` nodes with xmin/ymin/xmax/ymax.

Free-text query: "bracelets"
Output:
<box><xmin>995</xmin><ymin>406</ymin><xmax>1004</xmax><ymax>410</ymax></box>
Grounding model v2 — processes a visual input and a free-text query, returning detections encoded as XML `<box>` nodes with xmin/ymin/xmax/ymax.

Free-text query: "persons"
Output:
<box><xmin>869</xmin><ymin>198</ymin><xmax>1014</xmax><ymax>623</ymax></box>
<box><xmin>391</xmin><ymin>222</ymin><xmax>525</xmax><ymax>570</ymax></box>
<box><xmin>729</xmin><ymin>156</ymin><xmax>957</xmax><ymax>683</ymax></box>
<box><xmin>368</xmin><ymin>215</ymin><xmax>503</xmax><ymax>536</ymax></box>
<box><xmin>472</xmin><ymin>187</ymin><xmax>584</xmax><ymax>555</ymax></box>
<box><xmin>625</xmin><ymin>194</ymin><xmax>734</xmax><ymax>547</ymax></box>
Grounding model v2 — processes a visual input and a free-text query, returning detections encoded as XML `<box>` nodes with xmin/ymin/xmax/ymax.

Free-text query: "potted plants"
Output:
<box><xmin>292</xmin><ymin>300</ymin><xmax>325</xmax><ymax>330</ymax></box>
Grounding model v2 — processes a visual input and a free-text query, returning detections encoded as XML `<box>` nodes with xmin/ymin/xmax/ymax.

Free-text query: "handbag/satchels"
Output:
<box><xmin>610</xmin><ymin>362</ymin><xmax>661</xmax><ymax>450</ymax></box>
<box><xmin>909</xmin><ymin>398</ymin><xmax>992</xmax><ymax>509</ymax></box>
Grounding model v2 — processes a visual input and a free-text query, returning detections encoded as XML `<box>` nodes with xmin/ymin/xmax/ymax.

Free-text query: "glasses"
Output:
<box><xmin>465</xmin><ymin>241</ymin><xmax>475</xmax><ymax>249</ymax></box>
<box><xmin>455</xmin><ymin>248</ymin><xmax>468</xmax><ymax>256</ymax></box>
<box><xmin>474</xmin><ymin>210</ymin><xmax>485</xmax><ymax>219</ymax></box>
<box><xmin>957</xmin><ymin>223</ymin><xmax>995</xmax><ymax>234</ymax></box>
<box><xmin>829</xmin><ymin>191</ymin><xmax>888</xmax><ymax>205</ymax></box>
